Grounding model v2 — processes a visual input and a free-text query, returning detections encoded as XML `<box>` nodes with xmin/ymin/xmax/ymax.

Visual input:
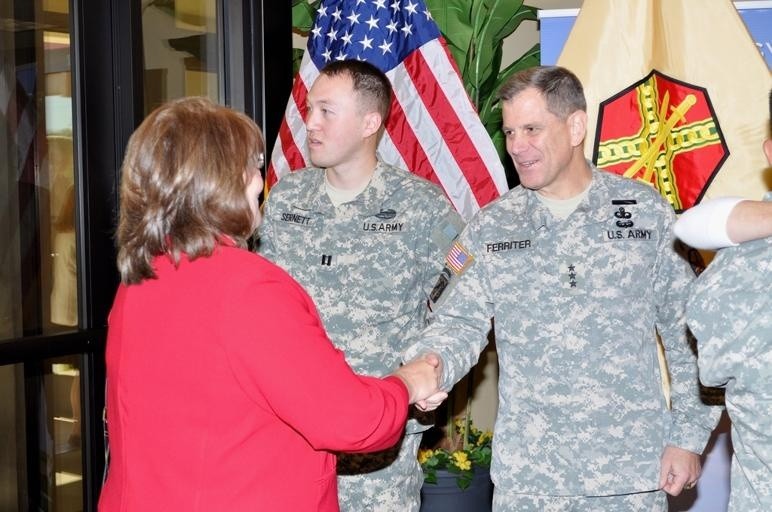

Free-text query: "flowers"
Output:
<box><xmin>417</xmin><ymin>417</ymin><xmax>493</xmax><ymax>491</ymax></box>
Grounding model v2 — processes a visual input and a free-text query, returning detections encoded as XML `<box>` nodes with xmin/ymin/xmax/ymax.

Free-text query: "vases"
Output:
<box><xmin>422</xmin><ymin>465</ymin><xmax>491</xmax><ymax>511</ymax></box>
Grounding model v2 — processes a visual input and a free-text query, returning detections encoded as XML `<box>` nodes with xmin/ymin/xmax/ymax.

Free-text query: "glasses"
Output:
<box><xmin>248</xmin><ymin>152</ymin><xmax>264</xmax><ymax>169</ymax></box>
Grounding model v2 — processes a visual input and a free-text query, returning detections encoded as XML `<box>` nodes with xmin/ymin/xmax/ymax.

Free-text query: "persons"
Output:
<box><xmin>97</xmin><ymin>95</ymin><xmax>444</xmax><ymax>512</ymax></box>
<box><xmin>681</xmin><ymin>88</ymin><xmax>772</xmax><ymax>511</ymax></box>
<box><xmin>670</xmin><ymin>196</ymin><xmax>772</xmax><ymax>252</ymax></box>
<box><xmin>249</xmin><ymin>55</ymin><xmax>466</xmax><ymax>511</ymax></box>
<box><xmin>403</xmin><ymin>62</ymin><xmax>712</xmax><ymax>512</ymax></box>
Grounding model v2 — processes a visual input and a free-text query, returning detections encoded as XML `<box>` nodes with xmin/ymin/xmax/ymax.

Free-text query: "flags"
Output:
<box><xmin>554</xmin><ymin>2</ymin><xmax>772</xmax><ymax>410</ymax></box>
<box><xmin>262</xmin><ymin>1</ymin><xmax>509</xmax><ymax>223</ymax></box>
